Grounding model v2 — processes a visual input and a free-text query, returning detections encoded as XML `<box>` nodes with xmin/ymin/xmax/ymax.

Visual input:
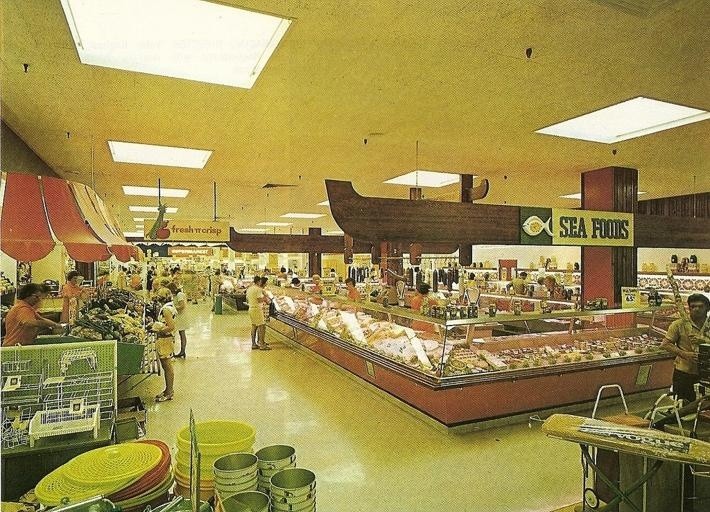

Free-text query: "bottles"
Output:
<box><xmin>514</xmin><ymin>303</ymin><xmax>521</xmax><ymax>315</ymax></box>
<box><xmin>420</xmin><ymin>301</ymin><xmax>479</xmax><ymax>319</ymax></box>
<box><xmin>586</xmin><ymin>297</ymin><xmax>608</xmax><ymax>310</ymax></box>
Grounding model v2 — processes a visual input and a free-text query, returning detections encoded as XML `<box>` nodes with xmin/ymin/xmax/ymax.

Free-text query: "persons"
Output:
<box><xmin>113</xmin><ymin>262</ymin><xmax>187</xmax><ymax>403</ymax></box>
<box><xmin>412</xmin><ymin>283</ymin><xmax>438</xmax><ymax>333</ymax></box>
<box><xmin>248</xmin><ymin>268</ymin><xmax>324</xmax><ymax>350</ymax></box>
<box><xmin>659</xmin><ymin>295</ymin><xmax>710</xmax><ymax>403</ymax></box>
<box><xmin>341</xmin><ymin>278</ymin><xmax>361</xmax><ymax>302</ymax></box>
<box><xmin>327</xmin><ymin>268</ymin><xmax>336</xmax><ymax>281</ymax></box>
<box><xmin>2</xmin><ymin>283</ymin><xmax>62</xmax><ymax>346</ymax></box>
<box><xmin>544</xmin><ymin>277</ymin><xmax>566</xmax><ymax>300</ymax></box>
<box><xmin>207</xmin><ymin>266</ymin><xmax>244</xmax><ymax>312</ymax></box>
<box><xmin>59</xmin><ymin>271</ymin><xmax>94</xmax><ymax>323</ymax></box>
<box><xmin>507</xmin><ymin>272</ymin><xmax>528</xmax><ymax>298</ymax></box>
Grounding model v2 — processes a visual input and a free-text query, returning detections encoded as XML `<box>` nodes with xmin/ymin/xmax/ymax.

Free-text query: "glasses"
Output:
<box><xmin>32</xmin><ymin>294</ymin><xmax>41</xmax><ymax>297</ymax></box>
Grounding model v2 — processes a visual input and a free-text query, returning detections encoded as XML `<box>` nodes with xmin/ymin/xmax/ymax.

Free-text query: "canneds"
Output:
<box><xmin>429</xmin><ymin>305</ymin><xmax>479</xmax><ymax>320</ymax></box>
<box><xmin>514</xmin><ymin>303</ymin><xmax>522</xmax><ymax>314</ymax></box>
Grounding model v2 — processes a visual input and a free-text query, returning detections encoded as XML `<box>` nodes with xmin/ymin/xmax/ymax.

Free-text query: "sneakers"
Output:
<box><xmin>252</xmin><ymin>341</ymin><xmax>271</xmax><ymax>349</ymax></box>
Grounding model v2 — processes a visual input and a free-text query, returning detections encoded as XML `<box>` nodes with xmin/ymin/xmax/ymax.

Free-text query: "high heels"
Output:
<box><xmin>156</xmin><ymin>392</ymin><xmax>173</xmax><ymax>402</ymax></box>
<box><xmin>175</xmin><ymin>353</ymin><xmax>185</xmax><ymax>358</ymax></box>
